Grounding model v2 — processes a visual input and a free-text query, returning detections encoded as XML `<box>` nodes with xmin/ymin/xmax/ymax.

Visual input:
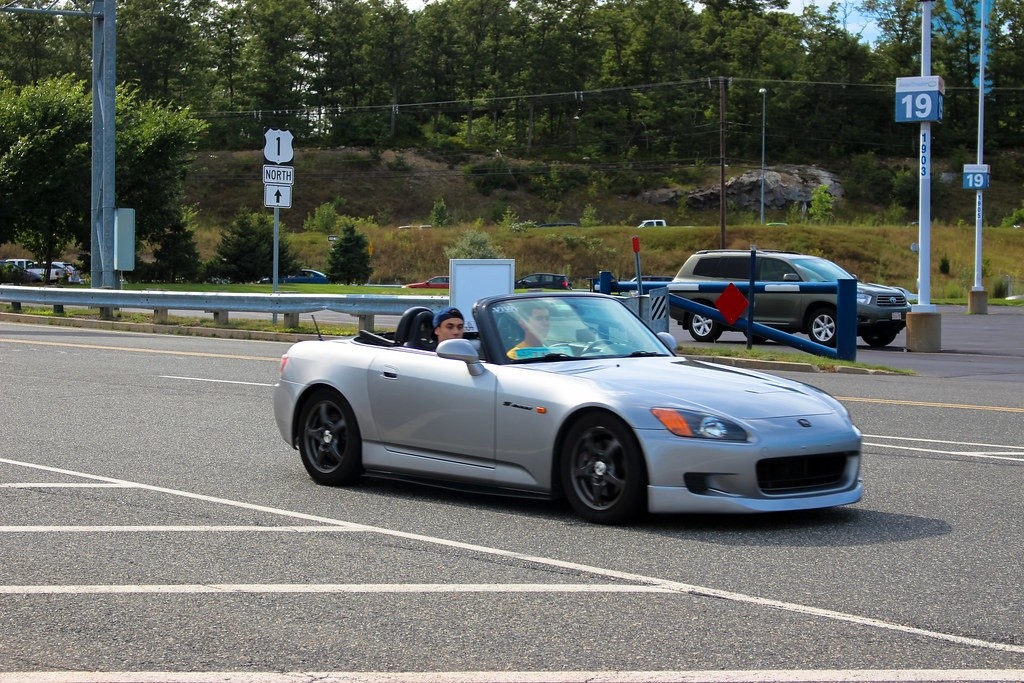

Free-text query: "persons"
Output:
<box><xmin>433</xmin><ymin>306</ymin><xmax>464</xmax><ymax>343</ymax></box>
<box><xmin>505</xmin><ymin>301</ymin><xmax>560</xmax><ymax>359</ymax></box>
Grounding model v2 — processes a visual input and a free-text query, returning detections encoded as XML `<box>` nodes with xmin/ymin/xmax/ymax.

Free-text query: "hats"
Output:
<box><xmin>429</xmin><ymin>306</ymin><xmax>463</xmax><ymax>340</ymax></box>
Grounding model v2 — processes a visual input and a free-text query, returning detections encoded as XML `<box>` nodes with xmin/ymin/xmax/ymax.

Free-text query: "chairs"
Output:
<box><xmin>496</xmin><ymin>311</ymin><xmax>525</xmax><ymax>354</ymax></box>
<box><xmin>394</xmin><ymin>306</ymin><xmax>440</xmax><ymax>352</ymax></box>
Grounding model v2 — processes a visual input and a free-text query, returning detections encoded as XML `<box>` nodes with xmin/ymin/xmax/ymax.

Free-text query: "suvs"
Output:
<box><xmin>669</xmin><ymin>247</ymin><xmax>913</xmax><ymax>348</ymax></box>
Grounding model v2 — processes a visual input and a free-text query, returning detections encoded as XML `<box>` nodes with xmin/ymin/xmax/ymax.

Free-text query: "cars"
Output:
<box><xmin>514</xmin><ymin>271</ymin><xmax>572</xmax><ymax>292</ymax></box>
<box><xmin>258</xmin><ymin>268</ymin><xmax>329</xmax><ymax>284</ymax></box>
<box><xmin>271</xmin><ymin>292</ymin><xmax>864</xmax><ymax>527</ymax></box>
<box><xmin>26</xmin><ymin>262</ymin><xmax>78</xmax><ymax>284</ymax></box>
<box><xmin>4</xmin><ymin>258</ymin><xmax>32</xmax><ymax>271</ymax></box>
<box><xmin>405</xmin><ymin>275</ymin><xmax>449</xmax><ymax>290</ymax></box>
<box><xmin>640</xmin><ymin>220</ymin><xmax>666</xmax><ymax>228</ymax></box>
